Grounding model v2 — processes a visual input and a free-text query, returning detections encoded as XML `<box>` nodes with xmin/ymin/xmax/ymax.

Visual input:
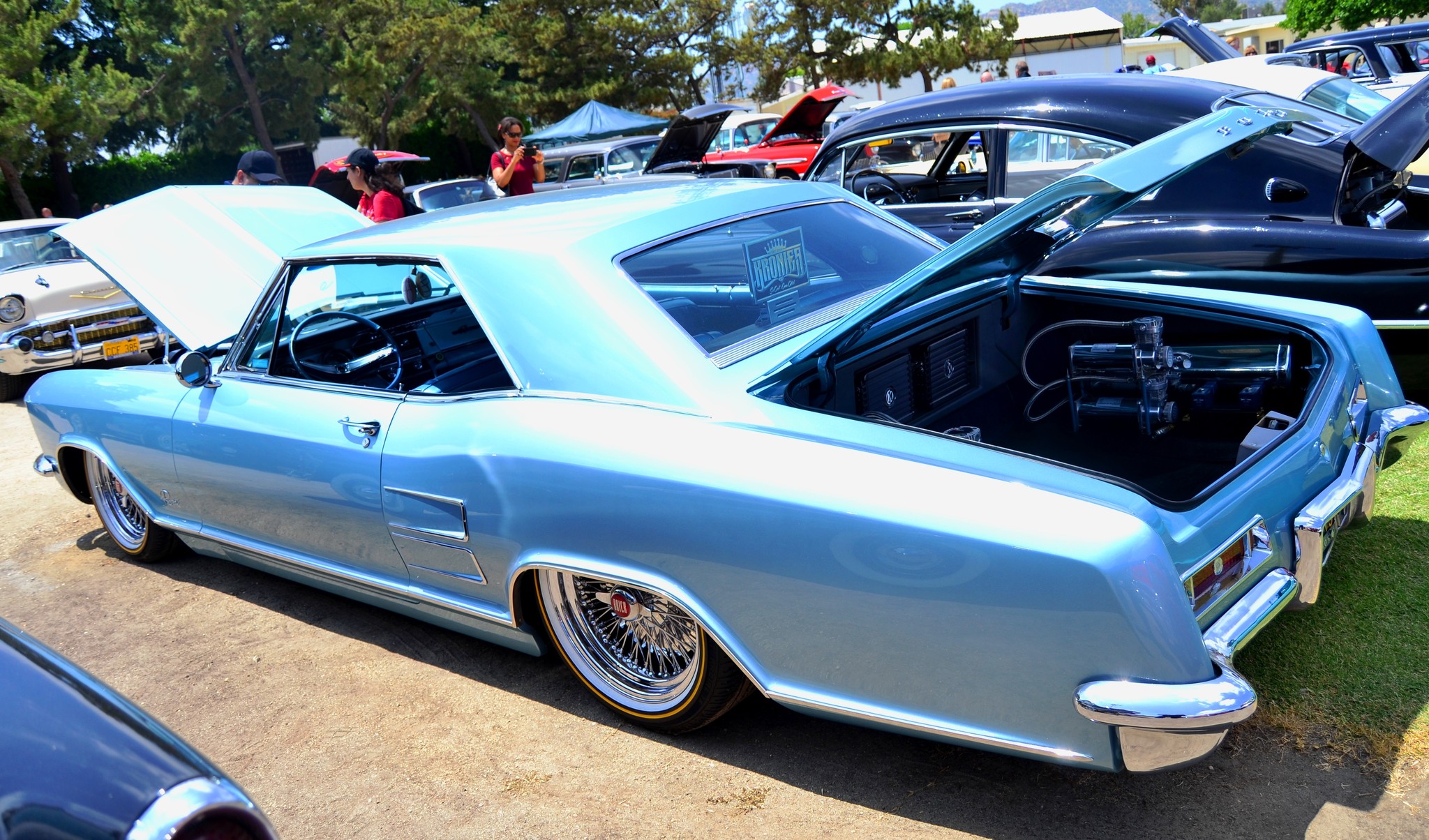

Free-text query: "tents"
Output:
<box><xmin>520</xmin><ymin>99</ymin><xmax>673</xmax><ymax>148</ymax></box>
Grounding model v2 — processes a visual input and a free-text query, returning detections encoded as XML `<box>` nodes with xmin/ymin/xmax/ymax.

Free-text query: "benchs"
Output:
<box><xmin>967</xmin><ymin>161</ymin><xmax>1093</xmax><ymax>203</ymax></box>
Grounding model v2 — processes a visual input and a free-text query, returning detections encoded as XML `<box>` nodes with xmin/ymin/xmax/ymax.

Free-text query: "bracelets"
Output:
<box><xmin>536</xmin><ymin>158</ymin><xmax>544</xmax><ymax>164</ymax></box>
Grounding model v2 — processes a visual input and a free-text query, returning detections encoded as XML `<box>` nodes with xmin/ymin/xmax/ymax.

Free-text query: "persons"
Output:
<box><xmin>981</xmin><ymin>72</ymin><xmax>993</xmax><ymax>83</ymax></box>
<box><xmin>343</xmin><ymin>147</ymin><xmax>404</xmax><ymax>224</ymax></box>
<box><xmin>758</xmin><ymin>125</ymin><xmax>767</xmax><ymax>142</ymax></box>
<box><xmin>932</xmin><ymin>77</ymin><xmax>968</xmax><ymax>155</ymax></box>
<box><xmin>454</xmin><ymin>175</ymin><xmax>471</xmax><ymax>202</ymax></box>
<box><xmin>42</xmin><ymin>208</ymin><xmax>53</xmax><ymax>217</ymax></box>
<box><xmin>91</xmin><ymin>203</ymin><xmax>102</xmax><ymax>213</ymax></box>
<box><xmin>1268</xmin><ymin>46</ymin><xmax>1278</xmax><ymax>54</ymax></box>
<box><xmin>1294</xmin><ymin>38</ymin><xmax>1318</xmax><ymax>69</ymax></box>
<box><xmin>1143</xmin><ymin>55</ymin><xmax>1167</xmax><ymax>75</ymax></box>
<box><xmin>490</xmin><ymin>117</ymin><xmax>545</xmax><ymax>196</ymax></box>
<box><xmin>225</xmin><ymin>149</ymin><xmax>285</xmax><ymax>186</ymax></box>
<box><xmin>1226</xmin><ymin>37</ymin><xmax>1240</xmax><ymax>51</ymax></box>
<box><xmin>1244</xmin><ymin>45</ymin><xmax>1258</xmax><ymax>57</ymax></box>
<box><xmin>1016</xmin><ymin>61</ymin><xmax>1032</xmax><ymax>78</ymax></box>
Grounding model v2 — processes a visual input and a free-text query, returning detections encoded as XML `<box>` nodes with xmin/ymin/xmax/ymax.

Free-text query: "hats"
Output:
<box><xmin>340</xmin><ymin>148</ymin><xmax>379</xmax><ymax>170</ymax></box>
<box><xmin>238</xmin><ymin>151</ymin><xmax>285</xmax><ymax>183</ymax></box>
<box><xmin>1146</xmin><ymin>55</ymin><xmax>1156</xmax><ymax>64</ymax></box>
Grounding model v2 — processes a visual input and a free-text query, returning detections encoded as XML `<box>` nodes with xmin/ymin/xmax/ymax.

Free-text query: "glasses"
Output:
<box><xmin>505</xmin><ymin>132</ymin><xmax>523</xmax><ymax>138</ymax></box>
<box><xmin>1247</xmin><ymin>51</ymin><xmax>1256</xmax><ymax>54</ymax></box>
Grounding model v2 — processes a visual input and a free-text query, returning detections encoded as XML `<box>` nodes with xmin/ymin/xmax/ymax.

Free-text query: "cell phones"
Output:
<box><xmin>523</xmin><ymin>148</ymin><xmax>536</xmax><ymax>156</ymax></box>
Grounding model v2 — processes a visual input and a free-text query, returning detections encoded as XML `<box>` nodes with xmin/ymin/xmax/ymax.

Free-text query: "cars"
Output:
<box><xmin>20</xmin><ymin>170</ymin><xmax>1427</xmax><ymax>780</ymax></box>
<box><xmin>0</xmin><ymin>9</ymin><xmax>1429</xmax><ymax>409</ymax></box>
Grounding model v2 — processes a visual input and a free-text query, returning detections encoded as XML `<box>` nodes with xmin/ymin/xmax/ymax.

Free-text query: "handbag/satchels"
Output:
<box><xmin>478</xmin><ymin>151</ymin><xmax>511</xmax><ymax>202</ymax></box>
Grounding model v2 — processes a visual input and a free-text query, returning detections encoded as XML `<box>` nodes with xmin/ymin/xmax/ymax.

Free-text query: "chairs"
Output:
<box><xmin>658</xmin><ymin>297</ymin><xmax>696</xmax><ymax>332</ymax></box>
<box><xmin>409</xmin><ymin>356</ymin><xmax>510</xmax><ymax>394</ymax></box>
